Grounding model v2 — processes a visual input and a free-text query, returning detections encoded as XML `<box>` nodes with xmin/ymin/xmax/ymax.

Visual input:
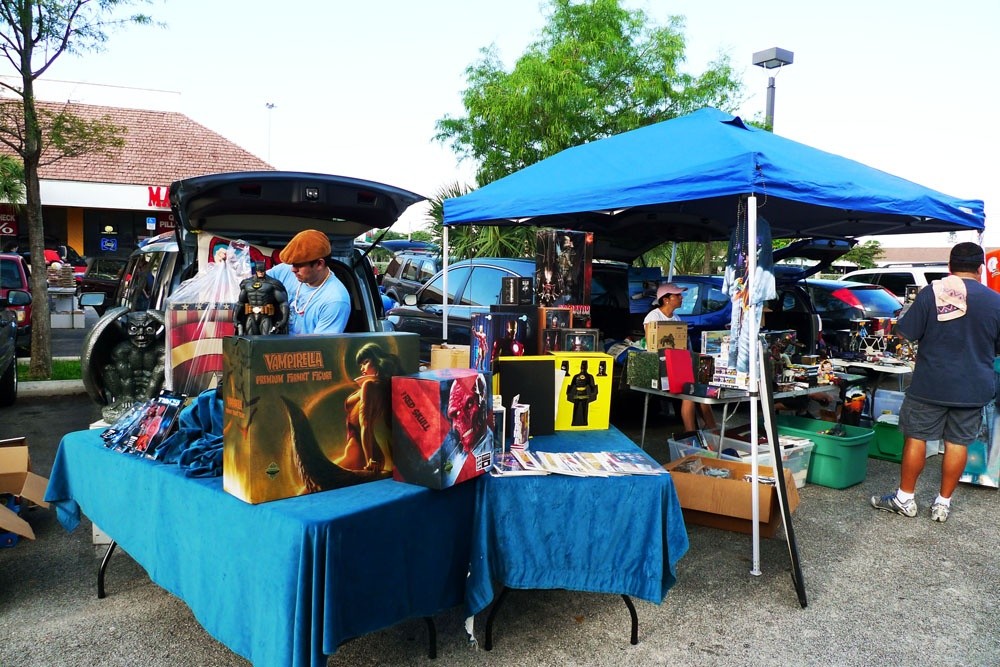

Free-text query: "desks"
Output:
<box><xmin>471</xmin><ymin>423</ymin><xmax>690</xmax><ymax>647</ymax></box>
<box><xmin>47</xmin><ymin>427</ymin><xmax>467</xmax><ymax>667</ymax></box>
<box><xmin>832</xmin><ymin>353</ymin><xmax>915</xmax><ymax>390</ymax></box>
<box><xmin>635</xmin><ymin>376</ymin><xmax>839</xmax><ymax>462</ymax></box>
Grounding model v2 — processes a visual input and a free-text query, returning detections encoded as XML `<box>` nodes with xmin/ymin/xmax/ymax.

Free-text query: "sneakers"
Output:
<box><xmin>871</xmin><ymin>489</ymin><xmax>918</xmax><ymax>518</ymax></box>
<box><xmin>929</xmin><ymin>501</ymin><xmax>950</xmax><ymax>522</ymax></box>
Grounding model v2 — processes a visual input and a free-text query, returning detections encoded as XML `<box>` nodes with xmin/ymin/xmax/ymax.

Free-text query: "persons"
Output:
<box><xmin>211</xmin><ymin>227</ymin><xmax>351</xmax><ymax>340</ymax></box>
<box><xmin>871</xmin><ymin>242</ymin><xmax>1000</xmax><ymax>524</ymax></box>
<box><xmin>641</xmin><ymin>281</ymin><xmax>718</xmax><ymax>434</ymax></box>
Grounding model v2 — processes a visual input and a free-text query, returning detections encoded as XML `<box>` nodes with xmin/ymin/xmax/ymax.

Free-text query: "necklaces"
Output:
<box><xmin>292</xmin><ymin>267</ymin><xmax>330</xmax><ymax>316</ymax></box>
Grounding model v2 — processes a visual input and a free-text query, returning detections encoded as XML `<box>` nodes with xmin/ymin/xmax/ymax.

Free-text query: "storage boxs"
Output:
<box><xmin>217</xmin><ymin>225</ymin><xmax>615</xmax><ymax>502</ymax></box>
<box><xmin>0</xmin><ymin>435</ymin><xmax>32</xmax><ymax>495</ymax></box>
<box><xmin>668</xmin><ymin>382</ymin><xmax>907</xmax><ymax>539</ymax></box>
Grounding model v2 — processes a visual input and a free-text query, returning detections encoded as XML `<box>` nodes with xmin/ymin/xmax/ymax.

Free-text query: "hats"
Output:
<box><xmin>657</xmin><ymin>284</ymin><xmax>688</xmax><ymax>300</ymax></box>
<box><xmin>278</xmin><ymin>229</ymin><xmax>332</xmax><ymax>264</ymax></box>
<box><xmin>949</xmin><ymin>249</ymin><xmax>985</xmax><ymax>264</ymax></box>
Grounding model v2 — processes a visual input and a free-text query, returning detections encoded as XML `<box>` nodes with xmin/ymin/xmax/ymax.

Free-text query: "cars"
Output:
<box><xmin>797</xmin><ymin>280</ymin><xmax>903</xmax><ymax>322</ymax></box>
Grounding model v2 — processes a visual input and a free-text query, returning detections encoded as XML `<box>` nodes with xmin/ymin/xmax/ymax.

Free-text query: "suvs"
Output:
<box><xmin>0</xmin><ymin>250</ymin><xmax>36</xmax><ymax>342</ymax></box>
<box><xmin>114</xmin><ymin>170</ymin><xmax>429</xmax><ymax>336</ymax></box>
<box><xmin>660</xmin><ymin>232</ymin><xmax>859</xmax><ymax>356</ymax></box>
<box><xmin>382</xmin><ymin>254</ymin><xmax>646</xmax><ymax>367</ymax></box>
<box><xmin>838</xmin><ymin>261</ymin><xmax>950</xmax><ymax>305</ymax></box>
<box><xmin>381</xmin><ymin>249</ymin><xmax>458</xmax><ymax>304</ymax></box>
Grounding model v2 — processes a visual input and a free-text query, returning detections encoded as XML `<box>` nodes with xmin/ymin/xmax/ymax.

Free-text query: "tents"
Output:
<box><xmin>438</xmin><ymin>107</ymin><xmax>994</xmax><ymax>585</ymax></box>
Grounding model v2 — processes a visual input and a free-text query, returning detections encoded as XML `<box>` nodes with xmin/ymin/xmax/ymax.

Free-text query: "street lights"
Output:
<box><xmin>751</xmin><ymin>47</ymin><xmax>794</xmax><ymax>132</ymax></box>
